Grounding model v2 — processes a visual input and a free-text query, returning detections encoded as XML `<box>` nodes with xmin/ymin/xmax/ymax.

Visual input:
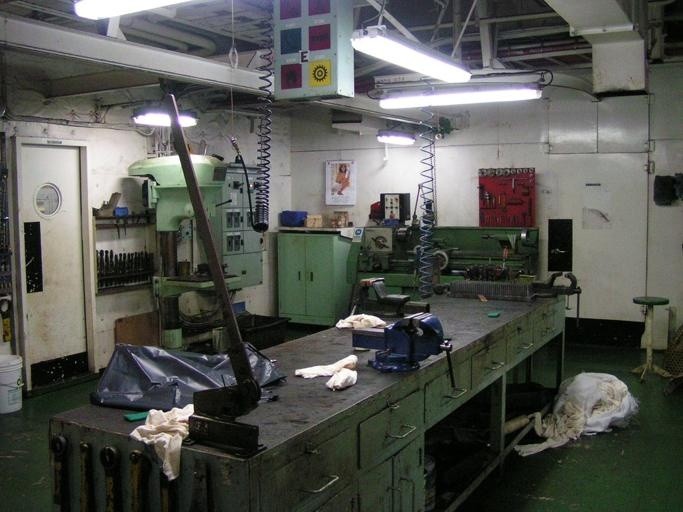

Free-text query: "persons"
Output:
<box><xmin>336</xmin><ymin>164</ymin><xmax>351</xmax><ymax>195</ymax></box>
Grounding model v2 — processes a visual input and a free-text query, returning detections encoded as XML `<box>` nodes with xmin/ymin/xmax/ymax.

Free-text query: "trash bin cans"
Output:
<box><xmin>236</xmin><ymin>314</ymin><xmax>292</xmax><ymax>351</ymax></box>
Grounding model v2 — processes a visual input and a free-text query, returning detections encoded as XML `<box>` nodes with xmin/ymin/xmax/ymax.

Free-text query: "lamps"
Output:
<box><xmin>74</xmin><ymin>1</ymin><xmax>185</xmax><ymax>21</ymax></box>
<box><xmin>374</xmin><ymin>119</ymin><xmax>418</xmax><ymax>146</ymax></box>
<box><xmin>348</xmin><ymin>0</ymin><xmax>544</xmax><ymax>110</ymax></box>
<box><xmin>131</xmin><ymin>104</ymin><xmax>199</xmax><ymax>129</ymax></box>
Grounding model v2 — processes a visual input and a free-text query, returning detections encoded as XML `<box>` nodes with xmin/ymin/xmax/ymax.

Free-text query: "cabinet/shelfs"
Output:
<box><xmin>276</xmin><ymin>230</ymin><xmax>362</xmax><ymax>326</ymax></box>
<box><xmin>48</xmin><ymin>296</ymin><xmax>566</xmax><ymax>512</ymax></box>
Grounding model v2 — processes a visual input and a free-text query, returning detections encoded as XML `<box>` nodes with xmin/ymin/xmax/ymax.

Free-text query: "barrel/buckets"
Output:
<box><xmin>0</xmin><ymin>354</ymin><xmax>24</xmax><ymax>415</ymax></box>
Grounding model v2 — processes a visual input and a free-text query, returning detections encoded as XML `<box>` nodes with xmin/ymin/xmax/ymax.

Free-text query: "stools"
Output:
<box><xmin>631</xmin><ymin>294</ymin><xmax>672</xmax><ymax>385</ymax></box>
